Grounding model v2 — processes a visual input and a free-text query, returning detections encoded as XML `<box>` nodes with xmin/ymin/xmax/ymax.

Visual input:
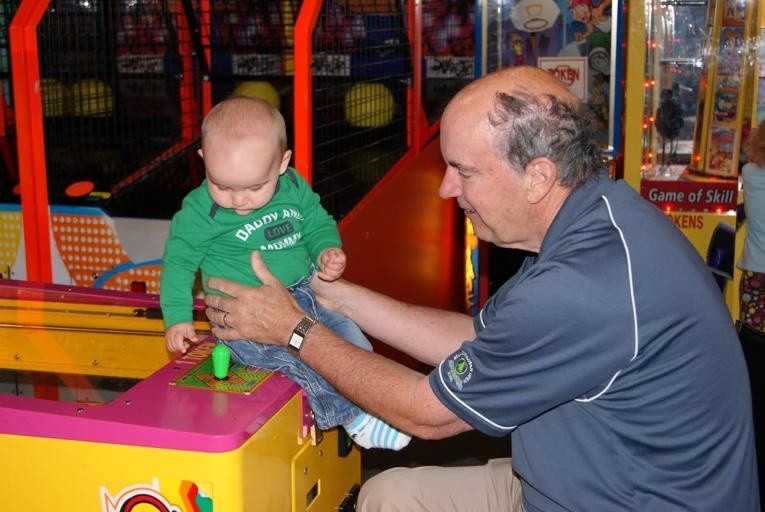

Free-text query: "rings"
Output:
<box><xmin>223</xmin><ymin>311</ymin><xmax>231</xmax><ymax>327</ymax></box>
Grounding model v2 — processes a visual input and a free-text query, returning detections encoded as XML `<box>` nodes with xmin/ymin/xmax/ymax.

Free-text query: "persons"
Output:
<box><xmin>159</xmin><ymin>92</ymin><xmax>415</xmax><ymax>454</ymax></box>
<box><xmin>739</xmin><ymin>115</ymin><xmax>764</xmax><ymax>337</ymax></box>
<box><xmin>205</xmin><ymin>63</ymin><xmax>763</xmax><ymax>512</ymax></box>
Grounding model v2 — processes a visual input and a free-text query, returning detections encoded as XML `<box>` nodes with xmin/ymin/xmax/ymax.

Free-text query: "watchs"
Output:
<box><xmin>288</xmin><ymin>316</ymin><xmax>319</xmax><ymax>361</ymax></box>
